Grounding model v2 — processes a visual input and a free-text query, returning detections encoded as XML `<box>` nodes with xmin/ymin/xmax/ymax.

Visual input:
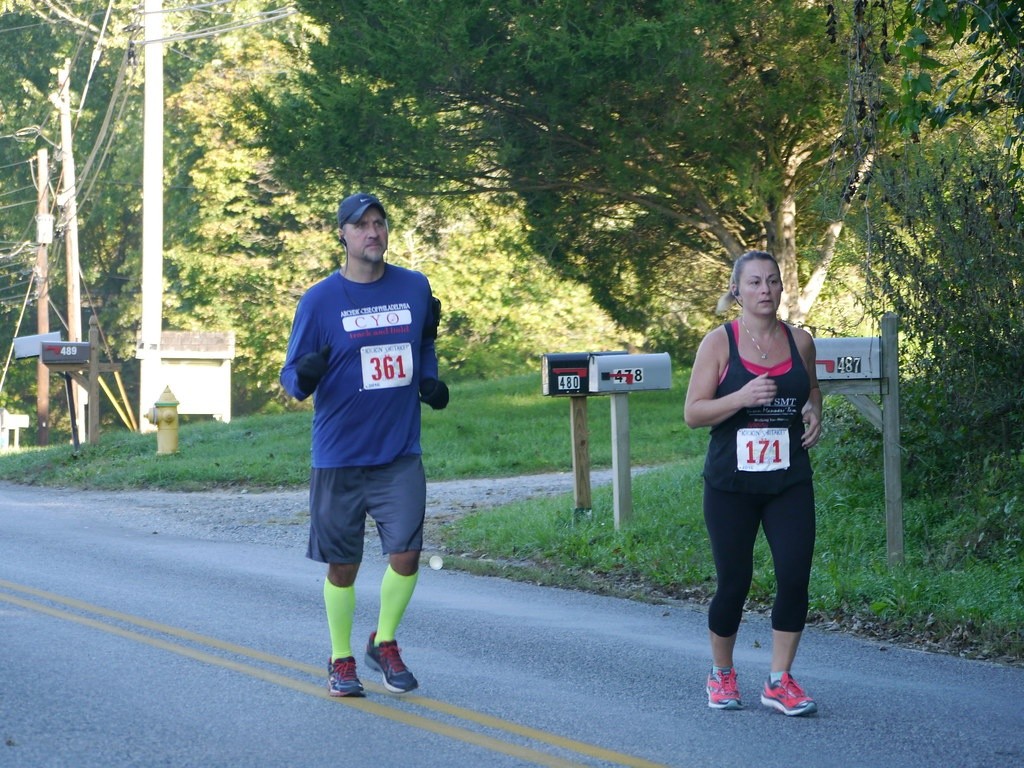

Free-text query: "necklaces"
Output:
<box><xmin>742</xmin><ymin>316</ymin><xmax>777</xmax><ymax>359</ymax></box>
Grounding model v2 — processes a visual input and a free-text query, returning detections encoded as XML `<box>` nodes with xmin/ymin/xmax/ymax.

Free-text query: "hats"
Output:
<box><xmin>337</xmin><ymin>194</ymin><xmax>385</xmax><ymax>228</ymax></box>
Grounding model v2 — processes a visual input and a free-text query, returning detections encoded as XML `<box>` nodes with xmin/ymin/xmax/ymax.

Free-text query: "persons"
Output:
<box><xmin>684</xmin><ymin>251</ymin><xmax>823</xmax><ymax>716</ymax></box>
<box><xmin>279</xmin><ymin>193</ymin><xmax>450</xmax><ymax>696</ymax></box>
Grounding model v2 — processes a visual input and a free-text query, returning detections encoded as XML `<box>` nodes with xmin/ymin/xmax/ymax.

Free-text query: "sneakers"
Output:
<box><xmin>706</xmin><ymin>670</ymin><xmax>741</xmax><ymax>710</ymax></box>
<box><xmin>327</xmin><ymin>656</ymin><xmax>363</xmax><ymax>696</ymax></box>
<box><xmin>363</xmin><ymin>631</ymin><xmax>418</xmax><ymax>692</ymax></box>
<box><xmin>761</xmin><ymin>671</ymin><xmax>818</xmax><ymax>717</ymax></box>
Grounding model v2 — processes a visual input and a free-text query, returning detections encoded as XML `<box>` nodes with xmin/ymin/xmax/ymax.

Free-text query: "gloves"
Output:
<box><xmin>419</xmin><ymin>377</ymin><xmax>450</xmax><ymax>410</ymax></box>
<box><xmin>297</xmin><ymin>345</ymin><xmax>332</xmax><ymax>393</ymax></box>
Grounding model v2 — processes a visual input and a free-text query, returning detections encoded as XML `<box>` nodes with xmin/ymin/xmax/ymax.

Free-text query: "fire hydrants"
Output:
<box><xmin>144</xmin><ymin>386</ymin><xmax>179</xmax><ymax>455</ymax></box>
